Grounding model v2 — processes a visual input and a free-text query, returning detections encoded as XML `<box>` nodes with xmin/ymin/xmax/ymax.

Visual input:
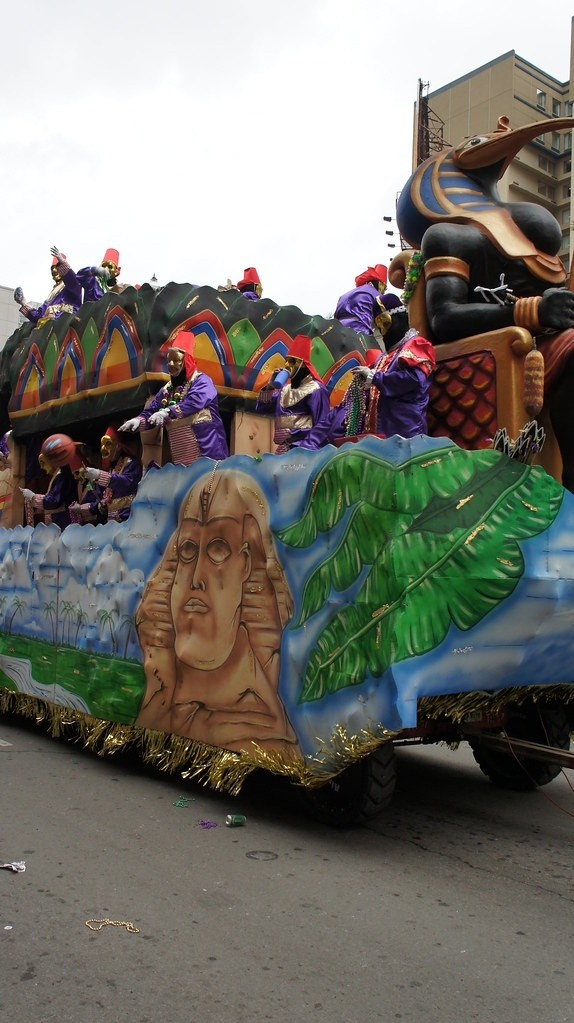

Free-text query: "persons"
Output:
<box><xmin>353</xmin><ymin>295</ymin><xmax>435</xmax><ymax>437</ymax></box>
<box><xmin>290</xmin><ymin>349</ymin><xmax>382</xmax><ymax>452</ymax></box>
<box><xmin>14</xmin><ymin>245</ymin><xmax>120</xmax><ymax>329</ymax></box>
<box><xmin>333</xmin><ymin>264</ymin><xmax>388</xmax><ymax>335</ymax></box>
<box><xmin>237</xmin><ymin>268</ymin><xmax>262</xmax><ymax>300</ymax></box>
<box><xmin>117</xmin><ymin>331</ymin><xmax>229</xmax><ymax>467</ymax></box>
<box><xmin>19</xmin><ymin>424</ymin><xmax>141</xmax><ymax>530</ymax></box>
<box><xmin>256</xmin><ymin>356</ymin><xmax>330</xmax><ymax>455</ymax></box>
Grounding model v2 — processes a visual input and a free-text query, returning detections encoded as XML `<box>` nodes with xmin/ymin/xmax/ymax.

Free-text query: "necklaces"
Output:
<box><xmin>68</xmin><ymin>501</ymin><xmax>82</xmax><ymax>525</ymax></box>
<box><xmin>160</xmin><ymin>381</ymin><xmax>185</xmax><ymax>409</ymax></box>
<box><xmin>345</xmin><ymin>371</ymin><xmax>370</xmax><ymax>436</ymax></box>
<box><xmin>25</xmin><ymin>499</ymin><xmax>34</xmax><ymax>527</ymax></box>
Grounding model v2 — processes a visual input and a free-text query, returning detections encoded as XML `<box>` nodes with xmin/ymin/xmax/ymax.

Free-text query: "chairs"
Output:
<box><xmin>388</xmin><ymin>249</ymin><xmax>563</xmax><ymax>487</ymax></box>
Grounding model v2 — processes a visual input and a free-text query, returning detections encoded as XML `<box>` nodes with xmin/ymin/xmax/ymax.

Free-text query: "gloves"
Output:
<box><xmin>21</xmin><ymin>489</ymin><xmax>34</xmax><ymax>499</ymax></box>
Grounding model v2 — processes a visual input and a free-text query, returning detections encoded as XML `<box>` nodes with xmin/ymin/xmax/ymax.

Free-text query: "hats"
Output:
<box><xmin>286</xmin><ymin>335</ymin><xmax>323</xmax><ymax>382</ymax></box>
<box><xmin>104</xmin><ymin>416</ymin><xmax>127</xmax><ymax>445</ymax></box>
<box><xmin>355</xmin><ymin>265</ymin><xmax>388</xmax><ymax>287</ymax></box>
<box><xmin>373</xmin><ymin>293</ymin><xmax>408</xmax><ymax>322</ymax></box>
<box><xmin>172</xmin><ymin>331</ymin><xmax>196</xmax><ymax>380</ymax></box>
<box><xmin>52</xmin><ymin>253</ymin><xmax>66</xmax><ymax>265</ymax></box>
<box><xmin>103</xmin><ymin>248</ymin><xmax>121</xmax><ymax>277</ymax></box>
<box><xmin>237</xmin><ymin>268</ymin><xmax>260</xmax><ymax>290</ymax></box>
